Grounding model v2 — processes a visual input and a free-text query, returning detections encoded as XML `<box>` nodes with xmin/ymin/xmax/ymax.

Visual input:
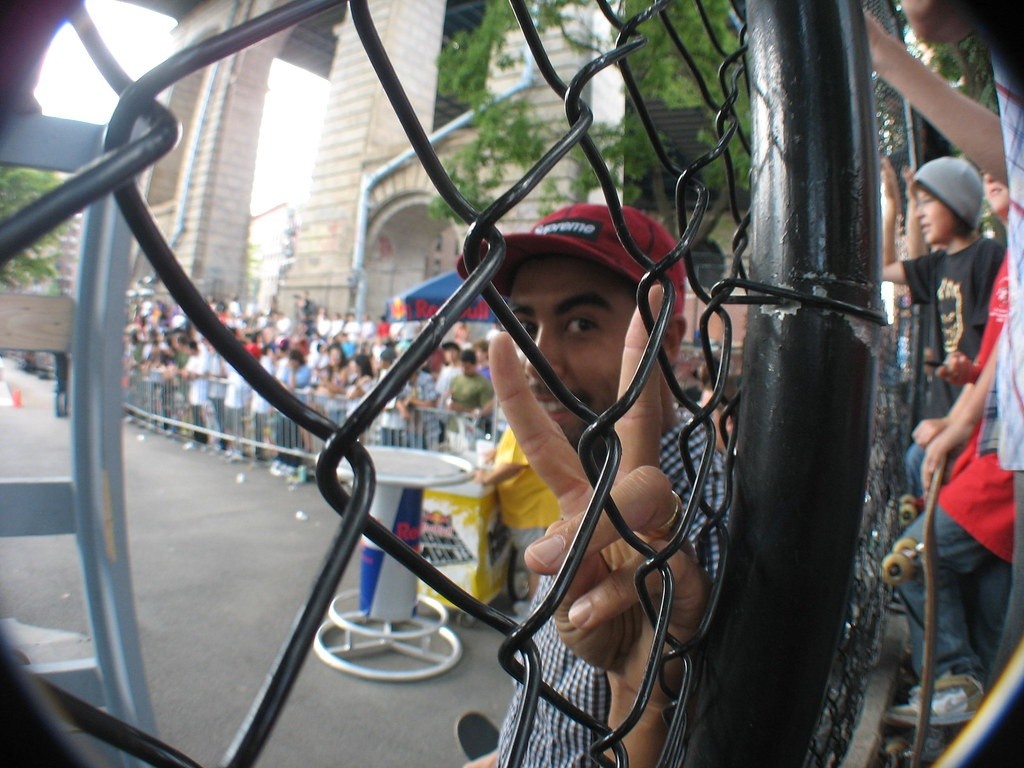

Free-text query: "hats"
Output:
<box><xmin>914</xmin><ymin>156</ymin><xmax>983</xmax><ymax>230</ymax></box>
<box><xmin>456</xmin><ymin>205</ymin><xmax>686</xmax><ymax>317</ymax></box>
<box><xmin>442</xmin><ymin>343</ymin><xmax>459</xmax><ymax>349</ymax></box>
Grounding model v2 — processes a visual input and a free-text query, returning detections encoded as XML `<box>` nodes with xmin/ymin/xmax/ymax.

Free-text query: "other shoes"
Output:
<box><xmin>270</xmin><ymin>459</ymin><xmax>296</xmax><ymax>475</ymax></box>
<box><xmin>183</xmin><ymin>440</ymin><xmax>243</xmax><ymax>465</ymax></box>
<box><xmin>510</xmin><ymin>600</ymin><xmax>530</xmax><ymax>625</ymax></box>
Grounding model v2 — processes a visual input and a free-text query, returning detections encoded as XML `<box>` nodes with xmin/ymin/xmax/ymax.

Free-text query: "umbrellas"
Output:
<box><xmin>383</xmin><ymin>270</ymin><xmax>505</xmax><ymax>322</ymax></box>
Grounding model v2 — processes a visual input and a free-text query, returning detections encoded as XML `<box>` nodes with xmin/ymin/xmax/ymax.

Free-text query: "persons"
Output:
<box><xmin>865</xmin><ymin>0</ymin><xmax>1022</xmax><ymax>760</ymax></box>
<box><xmin>458</xmin><ymin>203</ymin><xmax>729</xmax><ymax>768</ymax></box>
<box><xmin>23</xmin><ymin>296</ymin><xmax>740</xmax><ymax>627</ymax></box>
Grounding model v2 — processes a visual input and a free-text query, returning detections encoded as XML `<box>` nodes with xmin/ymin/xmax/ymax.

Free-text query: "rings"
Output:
<box><xmin>644</xmin><ymin>490</ymin><xmax>683</xmax><ymax>539</ymax></box>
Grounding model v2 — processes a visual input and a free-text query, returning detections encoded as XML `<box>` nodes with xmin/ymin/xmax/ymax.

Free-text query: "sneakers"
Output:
<box><xmin>882</xmin><ymin>675</ymin><xmax>984</xmax><ymax>729</ymax></box>
